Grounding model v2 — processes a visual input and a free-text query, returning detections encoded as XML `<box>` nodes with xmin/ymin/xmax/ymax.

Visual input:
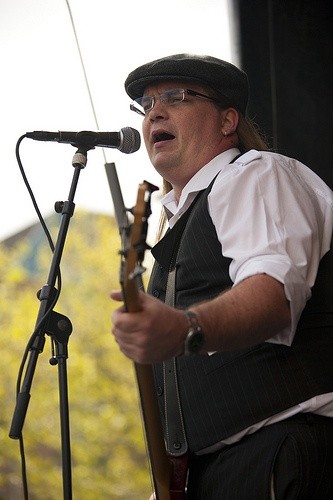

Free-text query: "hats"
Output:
<box><xmin>124</xmin><ymin>53</ymin><xmax>248</xmax><ymax>118</ymax></box>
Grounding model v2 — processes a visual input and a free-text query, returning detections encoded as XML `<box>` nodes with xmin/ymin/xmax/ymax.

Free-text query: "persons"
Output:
<box><xmin>110</xmin><ymin>55</ymin><xmax>333</xmax><ymax>500</ymax></box>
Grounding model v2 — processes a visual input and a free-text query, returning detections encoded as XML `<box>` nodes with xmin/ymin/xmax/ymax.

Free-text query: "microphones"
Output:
<box><xmin>26</xmin><ymin>126</ymin><xmax>141</xmax><ymax>154</ymax></box>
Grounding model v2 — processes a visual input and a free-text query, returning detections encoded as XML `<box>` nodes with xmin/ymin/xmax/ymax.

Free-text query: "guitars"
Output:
<box><xmin>119</xmin><ymin>182</ymin><xmax>191</xmax><ymax>500</ymax></box>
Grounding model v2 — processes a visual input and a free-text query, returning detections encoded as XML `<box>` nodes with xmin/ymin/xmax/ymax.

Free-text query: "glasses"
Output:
<box><xmin>129</xmin><ymin>87</ymin><xmax>228</xmax><ymax>115</ymax></box>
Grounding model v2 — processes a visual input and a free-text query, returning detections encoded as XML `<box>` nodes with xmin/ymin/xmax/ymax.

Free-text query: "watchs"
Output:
<box><xmin>184</xmin><ymin>310</ymin><xmax>204</xmax><ymax>357</ymax></box>
